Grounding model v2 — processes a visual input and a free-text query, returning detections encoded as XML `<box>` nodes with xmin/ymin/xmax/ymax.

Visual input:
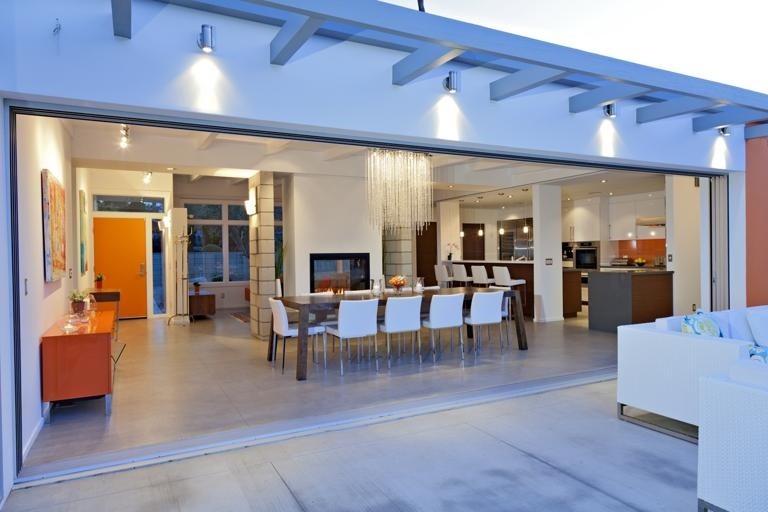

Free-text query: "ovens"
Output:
<box><xmin>571</xmin><ymin>242</ymin><xmax>600</xmax><ymax>288</ymax></box>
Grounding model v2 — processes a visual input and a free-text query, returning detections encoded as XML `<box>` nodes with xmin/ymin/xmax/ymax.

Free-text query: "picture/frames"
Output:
<box><xmin>41</xmin><ymin>170</ymin><xmax>90</xmax><ymax>284</ymax></box>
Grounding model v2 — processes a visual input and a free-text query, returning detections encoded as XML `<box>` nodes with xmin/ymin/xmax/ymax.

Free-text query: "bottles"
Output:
<box><xmin>446</xmin><ymin>243</ymin><xmax>457</xmax><ymax>260</ymax></box>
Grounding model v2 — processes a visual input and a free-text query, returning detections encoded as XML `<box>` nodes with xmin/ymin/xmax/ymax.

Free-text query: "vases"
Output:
<box><xmin>448</xmin><ymin>253</ymin><xmax>451</xmax><ymax>260</ymax></box>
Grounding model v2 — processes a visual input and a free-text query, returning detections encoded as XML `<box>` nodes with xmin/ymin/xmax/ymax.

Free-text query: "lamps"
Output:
<box><xmin>446</xmin><ymin>71</ymin><xmax>457</xmax><ymax>93</ymax></box>
<box><xmin>603</xmin><ymin>103</ymin><xmax>616</xmax><ymax>118</ymax></box>
<box><xmin>719</xmin><ymin>127</ymin><xmax>730</xmax><ymax>136</ymax></box>
<box><xmin>196</xmin><ymin>23</ymin><xmax>215</xmax><ymax>53</ymax></box>
<box><xmin>459</xmin><ymin>187</ymin><xmax>529</xmax><ymax>238</ymax></box>
<box><xmin>363</xmin><ymin>148</ymin><xmax>434</xmax><ymax>237</ymax></box>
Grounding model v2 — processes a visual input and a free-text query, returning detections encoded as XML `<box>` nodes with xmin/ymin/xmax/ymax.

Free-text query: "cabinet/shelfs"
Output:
<box><xmin>561</xmin><ymin>190</ymin><xmax>666</xmax><ymax>243</ymax></box>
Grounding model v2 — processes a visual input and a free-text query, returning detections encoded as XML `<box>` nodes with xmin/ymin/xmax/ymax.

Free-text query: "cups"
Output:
<box><xmin>655</xmin><ymin>255</ymin><xmax>666</xmax><ymax>266</ymax></box>
<box><xmin>412</xmin><ymin>277</ymin><xmax>425</xmax><ymax>293</ymax></box>
<box><xmin>370</xmin><ymin>278</ymin><xmax>383</xmax><ymax>297</ymax></box>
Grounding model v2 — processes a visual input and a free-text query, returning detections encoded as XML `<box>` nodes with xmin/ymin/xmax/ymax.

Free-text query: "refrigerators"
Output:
<box><xmin>497</xmin><ymin>218</ymin><xmax>534</xmax><ymax>261</ymax></box>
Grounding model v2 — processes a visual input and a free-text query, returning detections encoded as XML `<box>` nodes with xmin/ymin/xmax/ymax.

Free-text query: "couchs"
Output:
<box><xmin>696</xmin><ymin>362</ymin><xmax>768</xmax><ymax>512</ymax></box>
<box><xmin>615</xmin><ymin>306</ymin><xmax>768</xmax><ymax>445</ymax></box>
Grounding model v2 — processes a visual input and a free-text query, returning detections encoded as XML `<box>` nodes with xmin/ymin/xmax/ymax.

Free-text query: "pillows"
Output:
<box><xmin>681</xmin><ymin>309</ymin><xmax>723</xmax><ymax>337</ymax></box>
<box><xmin>746</xmin><ymin>342</ymin><xmax>768</xmax><ymax>361</ymax></box>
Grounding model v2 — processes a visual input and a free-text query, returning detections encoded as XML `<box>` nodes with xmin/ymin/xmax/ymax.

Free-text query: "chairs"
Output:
<box><xmin>268</xmin><ymin>263</ymin><xmax>526</xmax><ymax>377</ymax></box>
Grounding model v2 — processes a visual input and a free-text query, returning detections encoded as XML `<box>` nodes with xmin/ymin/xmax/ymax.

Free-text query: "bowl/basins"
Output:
<box><xmin>636</xmin><ymin>263</ymin><xmax>645</xmax><ymax>266</ymax></box>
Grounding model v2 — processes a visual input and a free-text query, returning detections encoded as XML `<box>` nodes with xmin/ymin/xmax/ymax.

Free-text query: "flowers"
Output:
<box><xmin>447</xmin><ymin>242</ymin><xmax>459</xmax><ymax>253</ymax></box>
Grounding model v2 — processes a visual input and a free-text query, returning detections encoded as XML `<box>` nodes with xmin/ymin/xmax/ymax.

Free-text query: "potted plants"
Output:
<box><xmin>67</xmin><ymin>289</ymin><xmax>89</xmax><ymax>314</ymax></box>
<box><xmin>93</xmin><ymin>271</ymin><xmax>105</xmax><ymax>290</ymax></box>
<box><xmin>275</xmin><ymin>238</ymin><xmax>288</xmax><ymax>297</ymax></box>
<box><xmin>193</xmin><ymin>281</ymin><xmax>201</xmax><ymax>292</ymax></box>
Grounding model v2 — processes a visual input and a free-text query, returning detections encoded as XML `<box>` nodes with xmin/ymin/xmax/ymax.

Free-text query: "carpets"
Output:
<box><xmin>227</xmin><ymin>310</ymin><xmax>250</xmax><ymax>324</ymax></box>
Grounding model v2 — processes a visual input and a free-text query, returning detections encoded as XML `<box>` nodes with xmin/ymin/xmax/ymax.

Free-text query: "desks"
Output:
<box><xmin>267</xmin><ymin>286</ymin><xmax>528</xmax><ymax>381</ymax></box>
<box><xmin>189</xmin><ymin>289</ymin><xmax>215</xmax><ymax>322</ymax></box>
<box><xmin>88</xmin><ymin>289</ymin><xmax>120</xmax><ymax>338</ymax></box>
<box><xmin>41</xmin><ymin>309</ymin><xmax>115</xmax><ymax>424</ymax></box>
